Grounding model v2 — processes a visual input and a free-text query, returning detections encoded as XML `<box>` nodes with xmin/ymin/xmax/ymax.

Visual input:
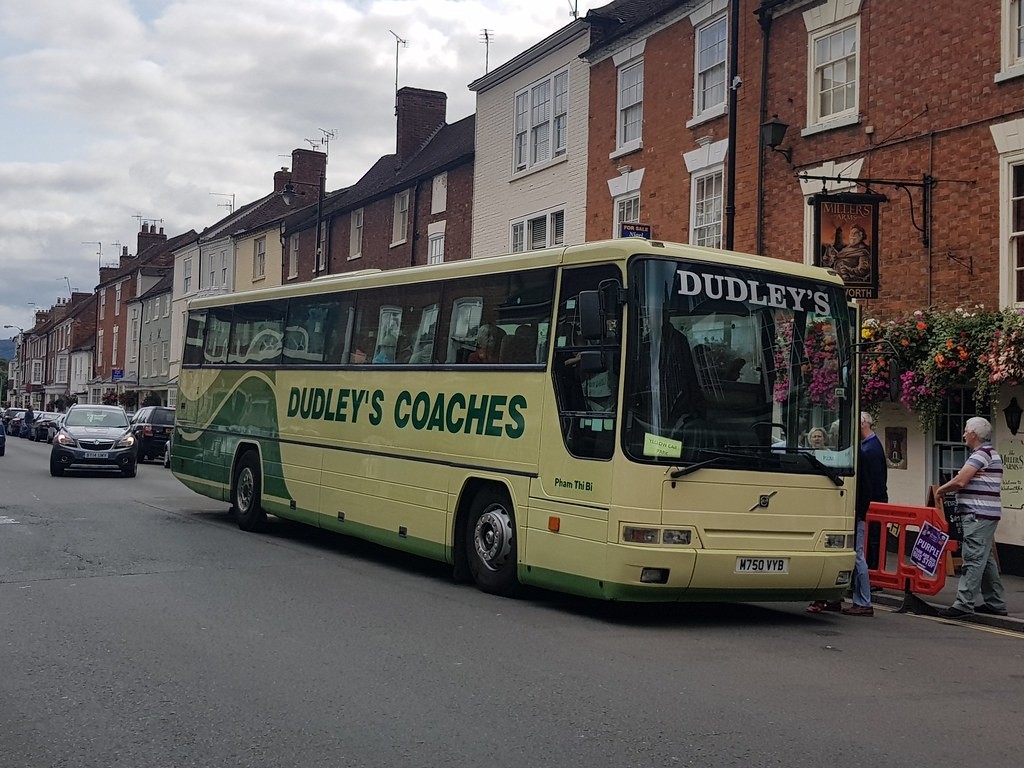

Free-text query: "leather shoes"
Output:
<box><xmin>807</xmin><ymin>601</ymin><xmax>841</xmax><ymax>611</ymax></box>
<box><xmin>842</xmin><ymin>604</ymin><xmax>875</xmax><ymax>617</ymax></box>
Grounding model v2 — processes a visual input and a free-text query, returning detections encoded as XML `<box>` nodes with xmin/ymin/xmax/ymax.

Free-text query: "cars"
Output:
<box><xmin>0</xmin><ymin>421</ymin><xmax>6</xmax><ymax>457</ymax></box>
<box><xmin>163</xmin><ymin>441</ymin><xmax>172</xmax><ymax>469</ymax></box>
<box><xmin>0</xmin><ymin>407</ymin><xmax>67</xmax><ymax>444</ymax></box>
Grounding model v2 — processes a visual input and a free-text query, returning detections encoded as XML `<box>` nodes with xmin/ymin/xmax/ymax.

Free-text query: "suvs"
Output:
<box><xmin>130</xmin><ymin>406</ymin><xmax>176</xmax><ymax>464</ymax></box>
<box><xmin>50</xmin><ymin>402</ymin><xmax>143</xmax><ymax>477</ymax></box>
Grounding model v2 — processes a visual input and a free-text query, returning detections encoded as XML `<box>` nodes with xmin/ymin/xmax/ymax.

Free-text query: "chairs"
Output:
<box><xmin>69</xmin><ymin>414</ymin><xmax>90</xmax><ymax>425</ymax></box>
<box><xmin>498</xmin><ymin>335</ymin><xmax>539</xmax><ymax>364</ymax></box>
<box><xmin>103</xmin><ymin>413</ymin><xmax>125</xmax><ymax>426</ymax></box>
<box><xmin>153</xmin><ymin>413</ymin><xmax>167</xmax><ymax>425</ymax></box>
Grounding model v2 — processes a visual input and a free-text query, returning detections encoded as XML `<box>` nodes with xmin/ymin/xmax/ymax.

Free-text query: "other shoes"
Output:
<box><xmin>975</xmin><ymin>604</ymin><xmax>1008</xmax><ymax>616</ymax></box>
<box><xmin>937</xmin><ymin>608</ymin><xmax>974</xmax><ymax>618</ymax></box>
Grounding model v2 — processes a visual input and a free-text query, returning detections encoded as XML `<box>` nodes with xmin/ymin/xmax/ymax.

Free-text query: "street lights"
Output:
<box><xmin>3</xmin><ymin>324</ymin><xmax>23</xmax><ymax>409</ymax></box>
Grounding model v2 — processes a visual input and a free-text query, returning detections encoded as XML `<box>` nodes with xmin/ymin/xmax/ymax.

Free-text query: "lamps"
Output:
<box><xmin>761</xmin><ymin>113</ymin><xmax>793</xmax><ymax>164</ymax></box>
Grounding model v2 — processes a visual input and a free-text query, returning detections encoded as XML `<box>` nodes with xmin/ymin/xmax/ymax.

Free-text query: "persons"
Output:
<box><xmin>935</xmin><ymin>417</ymin><xmax>1007</xmax><ymax>619</ymax></box>
<box><xmin>772</xmin><ymin>417</ymin><xmax>831</xmax><ymax>456</ymax></box>
<box><xmin>807</xmin><ymin>420</ymin><xmax>877</xmax><ymax>616</ymax></box>
<box><xmin>372</xmin><ymin>334</ymin><xmax>413</xmax><ymax>364</ymax></box>
<box><xmin>20</xmin><ymin>405</ymin><xmax>34</xmax><ymax>440</ymax></box>
<box><xmin>694</xmin><ymin>344</ymin><xmax>711</xmax><ymax>378</ymax></box>
<box><xmin>468</xmin><ymin>324</ymin><xmax>500</xmax><ymax>364</ymax></box>
<box><xmin>860</xmin><ymin>411</ymin><xmax>888</xmax><ymax>591</ymax></box>
<box><xmin>355</xmin><ymin>337</ymin><xmax>376</xmax><ymax>364</ymax></box>
<box><xmin>553</xmin><ymin>322</ymin><xmax>581</xmax><ymax>412</ymax></box>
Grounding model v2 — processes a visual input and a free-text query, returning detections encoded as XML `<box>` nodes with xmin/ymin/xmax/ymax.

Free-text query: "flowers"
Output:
<box><xmin>773</xmin><ymin>302</ymin><xmax>1024</xmax><ymax>438</ymax></box>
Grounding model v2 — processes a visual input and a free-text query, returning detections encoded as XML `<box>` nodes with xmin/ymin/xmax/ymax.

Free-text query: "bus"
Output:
<box><xmin>168</xmin><ymin>236</ymin><xmax>901</xmax><ymax>607</ymax></box>
